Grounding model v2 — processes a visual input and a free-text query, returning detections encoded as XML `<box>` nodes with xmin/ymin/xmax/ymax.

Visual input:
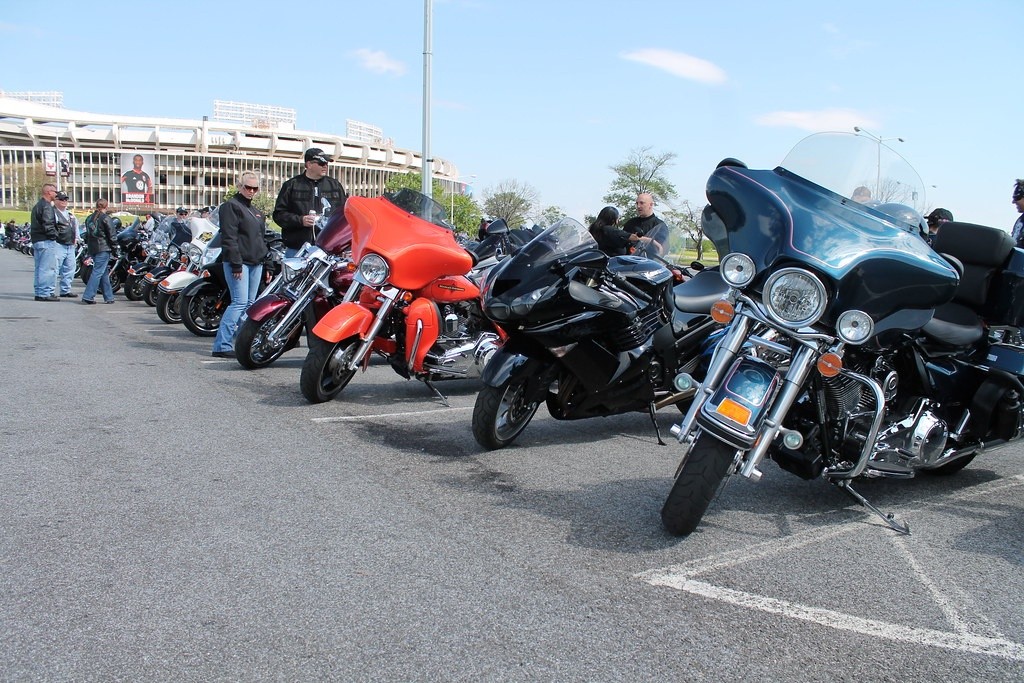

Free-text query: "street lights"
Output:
<box><xmin>895</xmin><ymin>179</ymin><xmax>938</xmax><ymax>212</ymax></box>
<box><xmin>16</xmin><ymin>122</ymin><xmax>96</xmax><ymax>196</ymax></box>
<box><xmin>855</xmin><ymin>126</ymin><xmax>904</xmax><ymax>199</ymax></box>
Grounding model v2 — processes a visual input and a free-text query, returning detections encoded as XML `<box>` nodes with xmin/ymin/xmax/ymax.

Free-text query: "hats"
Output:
<box><xmin>177</xmin><ymin>207</ymin><xmax>188</xmax><ymax>214</ymax></box>
<box><xmin>56</xmin><ymin>191</ymin><xmax>69</xmax><ymax>198</ymax></box>
<box><xmin>199</xmin><ymin>206</ymin><xmax>215</xmax><ymax>212</ymax></box>
<box><xmin>923</xmin><ymin>209</ymin><xmax>953</xmax><ymax>222</ymax></box>
<box><xmin>305</xmin><ymin>148</ymin><xmax>334</xmax><ymax>163</ymax></box>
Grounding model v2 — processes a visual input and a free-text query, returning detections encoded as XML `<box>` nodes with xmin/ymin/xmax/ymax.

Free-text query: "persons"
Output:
<box><xmin>920</xmin><ymin>208</ymin><xmax>953</xmax><ymax>244</ymax></box>
<box><xmin>590</xmin><ymin>193</ymin><xmax>670</xmax><ymax>262</ymax></box>
<box><xmin>211</xmin><ymin>170</ymin><xmax>273</xmax><ymax>358</ymax></box>
<box><xmin>0</xmin><ymin>181</ymin><xmax>217</xmax><ymax>305</ymax></box>
<box><xmin>851</xmin><ymin>186</ymin><xmax>874</xmax><ymax>207</ymax></box>
<box><xmin>1012</xmin><ymin>179</ymin><xmax>1024</xmax><ymax>249</ymax></box>
<box><xmin>121</xmin><ymin>154</ymin><xmax>153</xmax><ymax>203</ymax></box>
<box><xmin>272</xmin><ymin>148</ymin><xmax>347</xmax><ymax>348</ymax></box>
<box><xmin>61</xmin><ymin>154</ymin><xmax>69</xmax><ymax>173</ymax></box>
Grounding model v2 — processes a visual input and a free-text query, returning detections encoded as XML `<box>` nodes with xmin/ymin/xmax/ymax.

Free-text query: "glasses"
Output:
<box><xmin>179</xmin><ymin>214</ymin><xmax>187</xmax><ymax>216</ymax></box>
<box><xmin>314</xmin><ymin>161</ymin><xmax>328</xmax><ymax>166</ymax></box>
<box><xmin>244</xmin><ymin>185</ymin><xmax>258</xmax><ymax>192</ymax></box>
<box><xmin>56</xmin><ymin>198</ymin><xmax>68</xmax><ymax>201</ymax></box>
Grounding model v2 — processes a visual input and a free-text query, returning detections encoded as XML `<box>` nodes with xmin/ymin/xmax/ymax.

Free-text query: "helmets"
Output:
<box><xmin>112</xmin><ymin>217</ymin><xmax>122</xmax><ymax>229</ymax></box>
<box><xmin>479</xmin><ymin>220</ymin><xmax>494</xmax><ymax>240</ymax></box>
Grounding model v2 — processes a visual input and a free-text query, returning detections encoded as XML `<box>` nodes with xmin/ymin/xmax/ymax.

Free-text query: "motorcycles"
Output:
<box><xmin>662</xmin><ymin>159</ymin><xmax>1024</xmax><ymax>542</ymax></box>
<box><xmin>298</xmin><ymin>190</ymin><xmax>558</xmax><ymax>406</ymax></box>
<box><xmin>157</xmin><ymin>203</ymin><xmax>229</xmax><ymax>325</ymax></box>
<box><xmin>0</xmin><ymin>220</ymin><xmax>32</xmax><ymax>253</ymax></box>
<box><xmin>179</xmin><ymin>239</ymin><xmax>290</xmax><ymax>337</ymax></box>
<box><xmin>235</xmin><ymin>193</ymin><xmax>362</xmax><ymax>371</ymax></box>
<box><xmin>76</xmin><ymin>207</ymin><xmax>173</xmax><ymax>307</ymax></box>
<box><xmin>472</xmin><ymin>218</ymin><xmax>724</xmax><ymax>450</ymax></box>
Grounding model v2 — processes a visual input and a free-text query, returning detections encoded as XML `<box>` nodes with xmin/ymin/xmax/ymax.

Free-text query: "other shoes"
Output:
<box><xmin>60</xmin><ymin>292</ymin><xmax>78</xmax><ymax>297</ymax></box>
<box><xmin>81</xmin><ymin>298</ymin><xmax>96</xmax><ymax>304</ymax></box>
<box><xmin>107</xmin><ymin>301</ymin><xmax>114</xmax><ymax>303</ymax></box>
<box><xmin>35</xmin><ymin>294</ymin><xmax>60</xmax><ymax>301</ymax></box>
<box><xmin>212</xmin><ymin>350</ymin><xmax>238</xmax><ymax>358</ymax></box>
